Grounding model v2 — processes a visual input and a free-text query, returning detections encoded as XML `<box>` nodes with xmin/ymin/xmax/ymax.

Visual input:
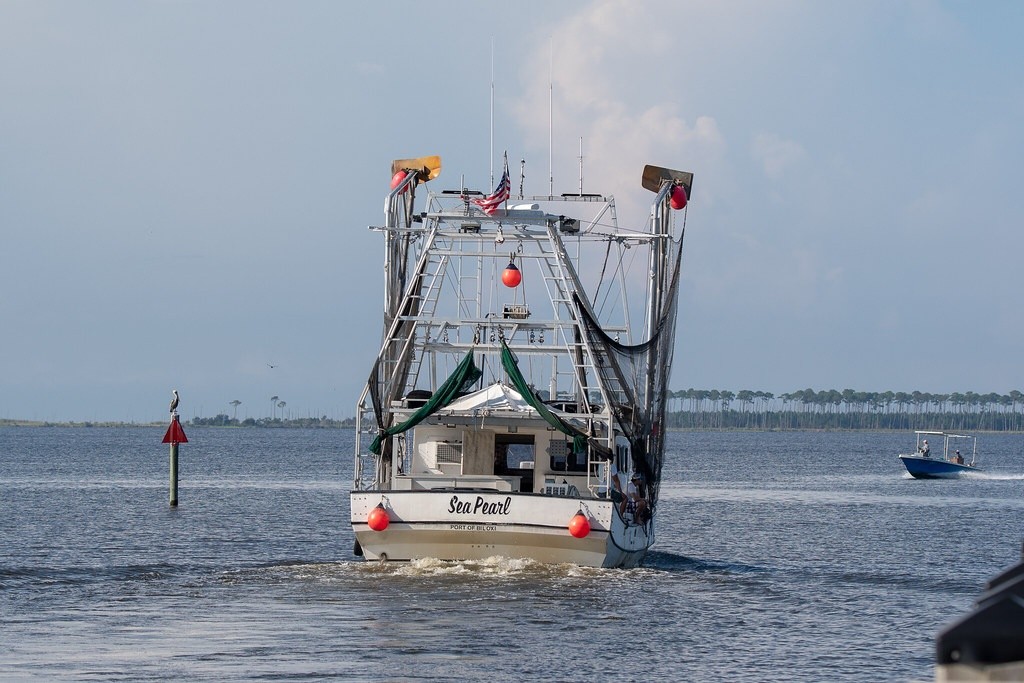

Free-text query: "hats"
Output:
<box><xmin>600</xmin><ymin>448</ymin><xmax>616</xmax><ymax>458</ymax></box>
<box><xmin>956</xmin><ymin>450</ymin><xmax>959</xmax><ymax>452</ymax></box>
<box><xmin>923</xmin><ymin>440</ymin><xmax>928</xmax><ymax>442</ymax></box>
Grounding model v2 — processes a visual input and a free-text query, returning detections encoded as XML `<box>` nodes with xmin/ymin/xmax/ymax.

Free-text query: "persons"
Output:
<box><xmin>567</xmin><ymin>443</ymin><xmax>577</xmax><ymax>466</ymax></box>
<box><xmin>919</xmin><ymin>439</ymin><xmax>964</xmax><ymax>463</ymax></box>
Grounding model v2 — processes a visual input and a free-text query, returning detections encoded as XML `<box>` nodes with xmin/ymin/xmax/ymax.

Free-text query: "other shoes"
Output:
<box><xmin>622</xmin><ymin>518</ymin><xmax>631</xmax><ymax>521</ymax></box>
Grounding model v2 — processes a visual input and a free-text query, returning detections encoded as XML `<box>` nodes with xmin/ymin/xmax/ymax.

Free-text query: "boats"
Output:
<box><xmin>347</xmin><ymin>83</ymin><xmax>693</xmax><ymax>574</ymax></box>
<box><xmin>898</xmin><ymin>430</ymin><xmax>985</xmax><ymax>481</ymax></box>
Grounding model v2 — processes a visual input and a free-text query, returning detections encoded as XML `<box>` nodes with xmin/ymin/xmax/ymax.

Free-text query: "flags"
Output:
<box><xmin>470</xmin><ymin>162</ymin><xmax>510</xmax><ymax>216</ymax></box>
